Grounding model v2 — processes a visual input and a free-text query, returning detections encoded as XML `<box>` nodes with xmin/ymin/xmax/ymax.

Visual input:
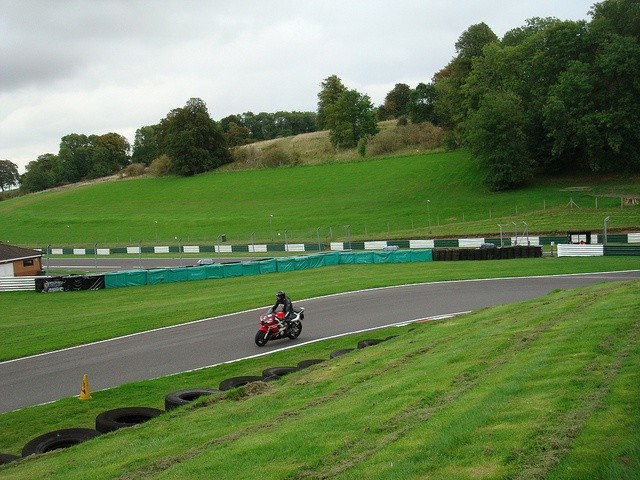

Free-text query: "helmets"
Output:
<box><xmin>276</xmin><ymin>291</ymin><xmax>285</xmax><ymax>301</ymax></box>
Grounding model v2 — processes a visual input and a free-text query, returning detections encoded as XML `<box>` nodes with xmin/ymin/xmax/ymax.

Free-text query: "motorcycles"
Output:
<box><xmin>255</xmin><ymin>307</ymin><xmax>304</xmax><ymax>347</ymax></box>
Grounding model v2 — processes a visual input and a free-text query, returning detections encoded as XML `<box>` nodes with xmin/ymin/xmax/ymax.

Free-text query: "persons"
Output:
<box><xmin>270</xmin><ymin>291</ymin><xmax>293</xmax><ymax>330</ymax></box>
